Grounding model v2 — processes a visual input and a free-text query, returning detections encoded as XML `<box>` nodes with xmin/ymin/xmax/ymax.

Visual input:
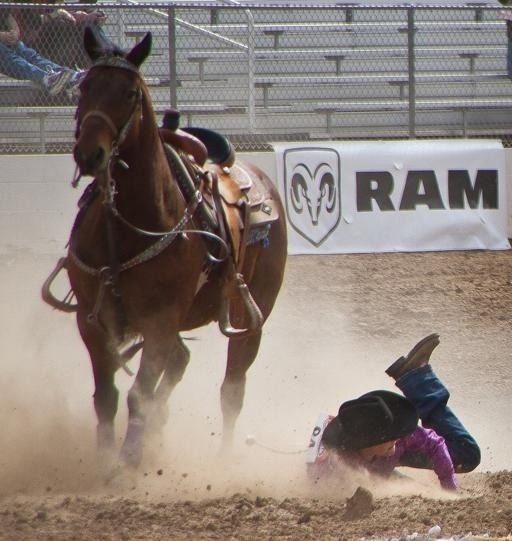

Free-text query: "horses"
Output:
<box><xmin>64</xmin><ymin>23</ymin><xmax>288</xmax><ymax>491</ymax></box>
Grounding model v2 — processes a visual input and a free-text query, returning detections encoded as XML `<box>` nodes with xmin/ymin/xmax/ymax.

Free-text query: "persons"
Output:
<box><xmin>302</xmin><ymin>331</ymin><xmax>481</xmax><ymax>496</ymax></box>
<box><xmin>1</xmin><ymin>0</ymin><xmax>110</xmax><ymax>96</ymax></box>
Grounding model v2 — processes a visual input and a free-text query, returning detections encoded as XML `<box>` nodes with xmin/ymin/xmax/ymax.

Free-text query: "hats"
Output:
<box><xmin>322</xmin><ymin>390</ymin><xmax>417</xmax><ymax>452</ymax></box>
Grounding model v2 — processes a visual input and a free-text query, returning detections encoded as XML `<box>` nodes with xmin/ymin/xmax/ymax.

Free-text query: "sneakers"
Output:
<box><xmin>64</xmin><ymin>70</ymin><xmax>89</xmax><ymax>94</ymax></box>
<box><xmin>44</xmin><ymin>69</ymin><xmax>65</xmax><ymax>95</ymax></box>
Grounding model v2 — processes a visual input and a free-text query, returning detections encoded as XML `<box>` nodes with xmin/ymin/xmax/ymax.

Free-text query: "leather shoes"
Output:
<box><xmin>385</xmin><ymin>332</ymin><xmax>440</xmax><ymax>381</ymax></box>
